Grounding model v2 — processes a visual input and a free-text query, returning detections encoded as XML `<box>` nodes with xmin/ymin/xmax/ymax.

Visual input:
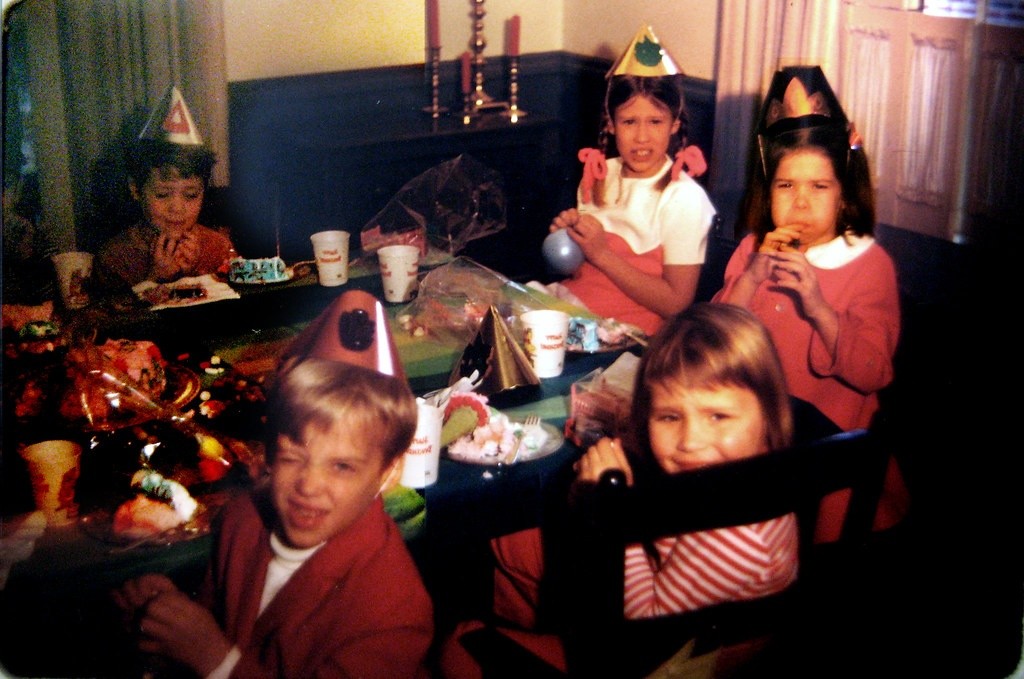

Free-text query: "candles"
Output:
<box><xmin>426</xmin><ymin>0</ymin><xmax>440</xmax><ymax>48</ymax></box>
<box><xmin>462</xmin><ymin>52</ymin><xmax>471</xmax><ymax>93</ymax></box>
<box><xmin>512</xmin><ymin>15</ymin><xmax>520</xmax><ymax>55</ymax></box>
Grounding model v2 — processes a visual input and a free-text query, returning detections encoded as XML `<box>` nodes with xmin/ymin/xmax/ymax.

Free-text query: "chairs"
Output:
<box><xmin>456</xmin><ymin>400</ymin><xmax>901</xmax><ymax>679</ymax></box>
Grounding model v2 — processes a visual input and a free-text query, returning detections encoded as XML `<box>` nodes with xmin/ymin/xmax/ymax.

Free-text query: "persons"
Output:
<box><xmin>543</xmin><ymin>23</ymin><xmax>716</xmax><ymax>334</ymax></box>
<box><xmin>98</xmin><ymin>83</ymin><xmax>236</xmax><ymax>286</ymax></box>
<box><xmin>709</xmin><ymin>113</ymin><xmax>901</xmax><ymax>433</ymax></box>
<box><xmin>114</xmin><ymin>289</ymin><xmax>435</xmax><ymax>679</ymax></box>
<box><xmin>491</xmin><ymin>302</ymin><xmax>799</xmax><ymax>631</ymax></box>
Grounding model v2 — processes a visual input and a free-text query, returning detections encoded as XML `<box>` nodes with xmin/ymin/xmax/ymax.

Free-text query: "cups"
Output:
<box><xmin>52</xmin><ymin>252</ymin><xmax>92</xmax><ymax>309</ymax></box>
<box><xmin>377</xmin><ymin>245</ymin><xmax>420</xmax><ymax>303</ymax></box>
<box><xmin>310</xmin><ymin>231</ymin><xmax>350</xmax><ymax>286</ymax></box>
<box><xmin>520</xmin><ymin>311</ymin><xmax>569</xmax><ymax>378</ymax></box>
<box><xmin>20</xmin><ymin>441</ymin><xmax>81</xmax><ymax>525</ymax></box>
<box><xmin>399</xmin><ymin>404</ymin><xmax>444</xmax><ymax>488</ymax></box>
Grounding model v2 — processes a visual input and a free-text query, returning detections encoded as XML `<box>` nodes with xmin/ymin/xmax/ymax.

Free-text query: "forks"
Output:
<box><xmin>505</xmin><ymin>415</ymin><xmax>539</xmax><ymax>465</ymax></box>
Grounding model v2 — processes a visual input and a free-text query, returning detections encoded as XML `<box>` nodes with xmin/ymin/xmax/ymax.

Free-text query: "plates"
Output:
<box><xmin>213</xmin><ymin>266</ymin><xmax>308</xmax><ymax>284</ymax></box>
<box><xmin>573</xmin><ymin>322</ymin><xmax>644</xmax><ymax>354</ymax></box>
<box><xmin>447</xmin><ymin>419</ymin><xmax>564</xmax><ymax>462</ymax></box>
<box><xmin>79</xmin><ymin>512</ymin><xmax>209</xmax><ymax>545</ymax></box>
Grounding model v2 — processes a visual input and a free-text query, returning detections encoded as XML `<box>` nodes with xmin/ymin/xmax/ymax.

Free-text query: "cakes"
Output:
<box><xmin>56</xmin><ymin>340</ymin><xmax>168</xmax><ymax>419</ymax></box>
<box><xmin>225</xmin><ymin>256</ymin><xmax>291</xmax><ymax>281</ymax></box>
<box><xmin>128</xmin><ymin>469</ymin><xmax>199</xmax><ymax>534</ymax></box>
<box><xmin>566</xmin><ymin>316</ymin><xmax>601</xmax><ymax>350</ymax></box>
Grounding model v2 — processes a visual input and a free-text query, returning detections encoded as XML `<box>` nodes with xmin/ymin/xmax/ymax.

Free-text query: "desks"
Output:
<box><xmin>0</xmin><ymin>246</ymin><xmax>650</xmax><ymax>678</ymax></box>
